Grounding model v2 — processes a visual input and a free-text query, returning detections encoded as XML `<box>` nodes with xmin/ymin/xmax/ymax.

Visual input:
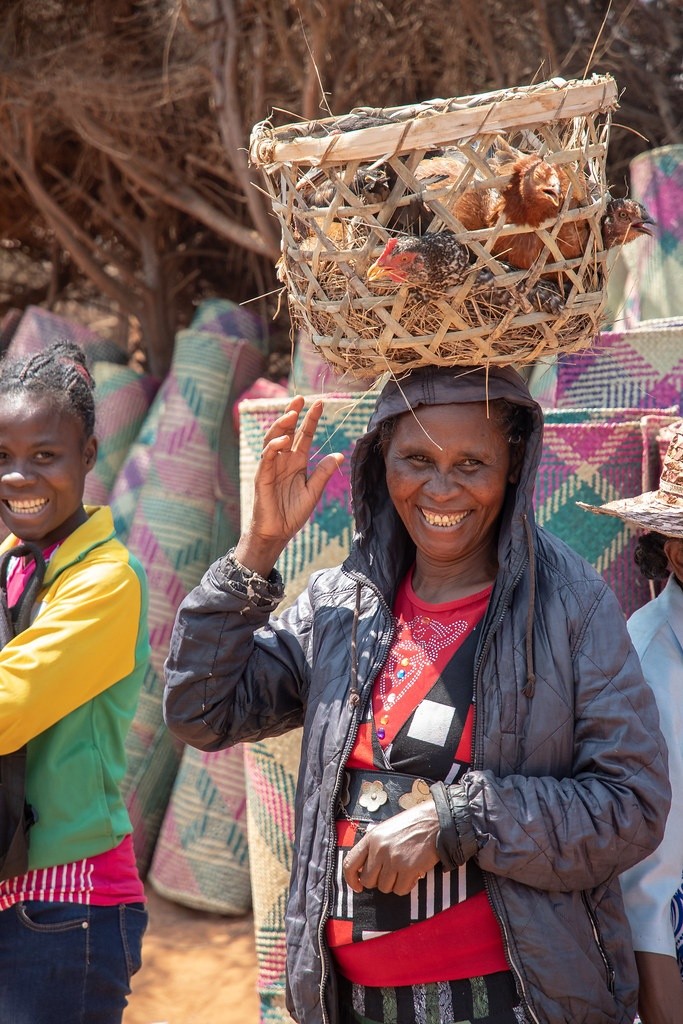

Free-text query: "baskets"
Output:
<box><xmin>250</xmin><ymin>74</ymin><xmax>618</xmax><ymax>378</ymax></box>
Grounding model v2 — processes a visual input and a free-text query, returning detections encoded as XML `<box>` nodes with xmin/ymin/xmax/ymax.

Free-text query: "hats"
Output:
<box><xmin>576</xmin><ymin>424</ymin><xmax>683</xmax><ymax>539</ymax></box>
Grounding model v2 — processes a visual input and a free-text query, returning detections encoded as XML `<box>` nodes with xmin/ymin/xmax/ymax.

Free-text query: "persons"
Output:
<box><xmin>0</xmin><ymin>340</ymin><xmax>151</xmax><ymax>1024</ymax></box>
<box><xmin>576</xmin><ymin>428</ymin><xmax>683</xmax><ymax>1024</ymax></box>
<box><xmin>163</xmin><ymin>366</ymin><xmax>672</xmax><ymax>1024</ymax></box>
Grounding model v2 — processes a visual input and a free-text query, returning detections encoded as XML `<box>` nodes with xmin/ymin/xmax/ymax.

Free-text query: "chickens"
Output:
<box><xmin>290</xmin><ymin>146</ymin><xmax>655</xmax><ymax>303</ymax></box>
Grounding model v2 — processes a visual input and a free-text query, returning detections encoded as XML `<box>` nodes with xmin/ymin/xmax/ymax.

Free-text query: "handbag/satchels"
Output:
<box><xmin>0</xmin><ymin>544</ymin><xmax>45</xmax><ymax>882</ymax></box>
